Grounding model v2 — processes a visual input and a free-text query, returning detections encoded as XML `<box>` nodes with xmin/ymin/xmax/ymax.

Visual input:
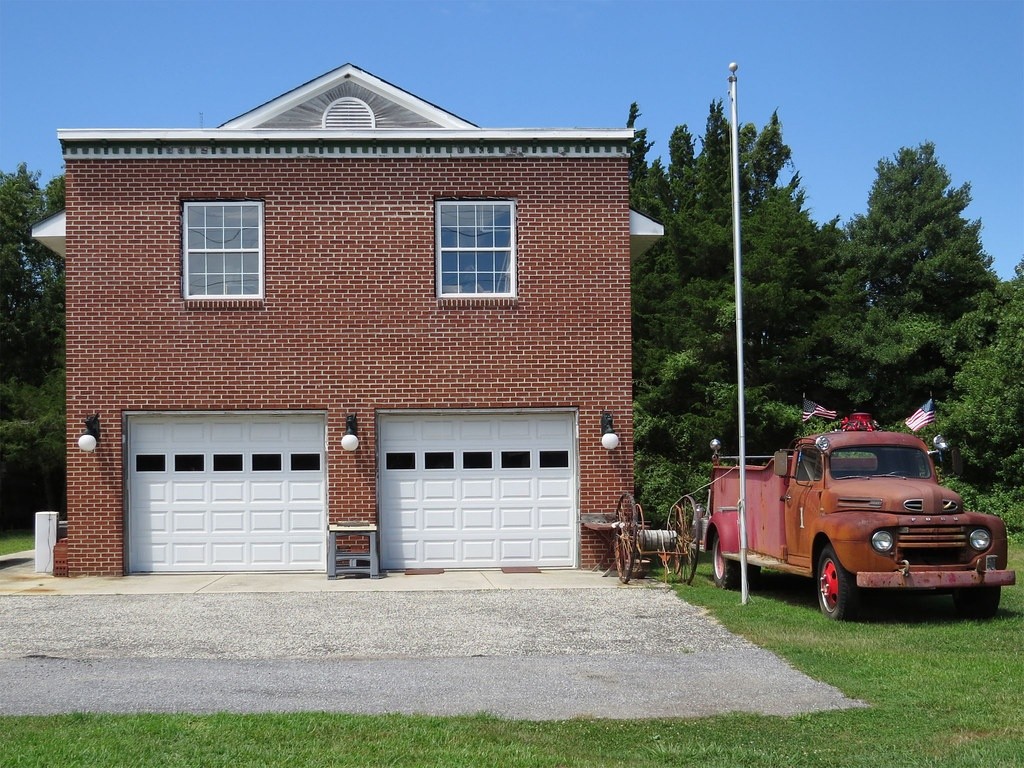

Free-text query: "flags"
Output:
<box><xmin>803</xmin><ymin>400</ymin><xmax>837</xmax><ymax>421</ymax></box>
<box><xmin>905</xmin><ymin>399</ymin><xmax>935</xmax><ymax>432</ymax></box>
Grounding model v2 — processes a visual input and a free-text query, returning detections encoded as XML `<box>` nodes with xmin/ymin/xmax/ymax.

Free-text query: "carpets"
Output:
<box><xmin>502</xmin><ymin>567</ymin><xmax>541</xmax><ymax>573</ymax></box>
<box><xmin>404</xmin><ymin>568</ymin><xmax>444</xmax><ymax>575</ymax></box>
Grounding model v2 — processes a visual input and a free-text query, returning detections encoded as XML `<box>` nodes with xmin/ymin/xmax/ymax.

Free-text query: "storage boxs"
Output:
<box><xmin>53</xmin><ymin>538</ymin><xmax>69</xmax><ymax>578</ymax></box>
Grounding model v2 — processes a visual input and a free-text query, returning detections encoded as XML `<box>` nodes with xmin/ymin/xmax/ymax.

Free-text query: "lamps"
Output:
<box><xmin>341</xmin><ymin>410</ymin><xmax>359</xmax><ymax>451</ymax></box>
<box><xmin>78</xmin><ymin>413</ymin><xmax>102</xmax><ymax>451</ymax></box>
<box><xmin>601</xmin><ymin>407</ymin><xmax>619</xmax><ymax>449</ymax></box>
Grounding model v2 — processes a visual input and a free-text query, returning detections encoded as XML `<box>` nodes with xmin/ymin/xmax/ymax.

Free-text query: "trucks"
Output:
<box><xmin>690</xmin><ymin>412</ymin><xmax>1016</xmax><ymax>621</ymax></box>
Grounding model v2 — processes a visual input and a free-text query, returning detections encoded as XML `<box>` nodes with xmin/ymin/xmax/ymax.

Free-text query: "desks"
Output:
<box><xmin>584</xmin><ymin>523</ymin><xmax>650</xmax><ymax>578</ymax></box>
<box><xmin>328</xmin><ymin>524</ymin><xmax>380</xmax><ymax>580</ymax></box>
